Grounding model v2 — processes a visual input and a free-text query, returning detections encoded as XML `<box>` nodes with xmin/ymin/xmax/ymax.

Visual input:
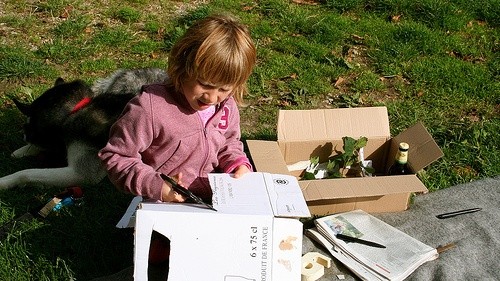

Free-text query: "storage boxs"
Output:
<box><xmin>246</xmin><ymin>107</ymin><xmax>444</xmax><ymax>217</ymax></box>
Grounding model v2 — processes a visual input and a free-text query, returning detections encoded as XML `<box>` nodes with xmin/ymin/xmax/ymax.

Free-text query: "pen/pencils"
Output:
<box><xmin>436</xmin><ymin>207</ymin><xmax>482</xmax><ymax>219</ymax></box>
<box><xmin>336</xmin><ymin>234</ymin><xmax>386</xmax><ymax>249</ymax></box>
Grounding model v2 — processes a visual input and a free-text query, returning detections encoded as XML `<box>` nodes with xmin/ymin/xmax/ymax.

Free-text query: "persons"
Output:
<box><xmin>97</xmin><ymin>15</ymin><xmax>256</xmax><ymax>265</ymax></box>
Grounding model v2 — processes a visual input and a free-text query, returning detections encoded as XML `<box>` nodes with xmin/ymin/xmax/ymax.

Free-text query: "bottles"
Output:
<box><xmin>387</xmin><ymin>142</ymin><xmax>413</xmax><ymax>176</ymax></box>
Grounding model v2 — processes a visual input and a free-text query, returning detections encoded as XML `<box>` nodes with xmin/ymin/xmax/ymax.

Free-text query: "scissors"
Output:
<box><xmin>160</xmin><ymin>173</ymin><xmax>217</xmax><ymax>212</ymax></box>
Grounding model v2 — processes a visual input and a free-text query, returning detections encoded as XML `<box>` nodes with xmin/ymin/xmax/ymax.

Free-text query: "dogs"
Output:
<box><xmin>0</xmin><ymin>67</ymin><xmax>169</xmax><ymax>190</ymax></box>
